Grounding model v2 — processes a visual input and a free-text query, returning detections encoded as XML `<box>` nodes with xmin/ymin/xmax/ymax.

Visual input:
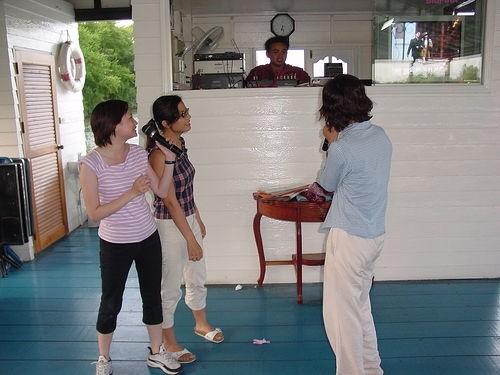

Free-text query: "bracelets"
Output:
<box><xmin>165</xmin><ymin>161</ymin><xmax>176</xmax><ymax>164</ymax></box>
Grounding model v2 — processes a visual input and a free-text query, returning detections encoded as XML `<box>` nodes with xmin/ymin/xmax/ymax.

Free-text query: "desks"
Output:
<box><xmin>253</xmin><ymin>194</ymin><xmax>337</xmax><ymax>304</ymax></box>
<box><xmin>0</xmin><ymin>157</ymin><xmax>33</xmax><ymax>278</ymax></box>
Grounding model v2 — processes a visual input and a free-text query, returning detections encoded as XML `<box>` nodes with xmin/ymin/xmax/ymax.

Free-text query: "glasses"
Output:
<box><xmin>180</xmin><ymin>108</ymin><xmax>189</xmax><ymax>118</ymax></box>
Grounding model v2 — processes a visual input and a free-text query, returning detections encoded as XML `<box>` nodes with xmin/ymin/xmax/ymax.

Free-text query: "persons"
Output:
<box><xmin>244</xmin><ymin>36</ymin><xmax>311</xmax><ymax>88</ymax></box>
<box><xmin>316</xmin><ymin>74</ymin><xmax>392</xmax><ymax>375</ymax></box>
<box><xmin>146</xmin><ymin>95</ymin><xmax>225</xmax><ymax>364</ymax></box>
<box><xmin>406</xmin><ymin>30</ymin><xmax>434</xmax><ymax>60</ymax></box>
<box><xmin>77</xmin><ymin>100</ymin><xmax>184</xmax><ymax>375</ymax></box>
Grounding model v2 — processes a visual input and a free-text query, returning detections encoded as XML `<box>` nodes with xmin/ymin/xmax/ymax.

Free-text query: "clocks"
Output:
<box><xmin>271</xmin><ymin>13</ymin><xmax>295</xmax><ymax>37</ymax></box>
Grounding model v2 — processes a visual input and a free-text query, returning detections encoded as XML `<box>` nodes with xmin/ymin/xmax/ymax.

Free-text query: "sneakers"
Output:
<box><xmin>91</xmin><ymin>356</ymin><xmax>112</xmax><ymax>375</ymax></box>
<box><xmin>147</xmin><ymin>343</ymin><xmax>184</xmax><ymax>375</ymax></box>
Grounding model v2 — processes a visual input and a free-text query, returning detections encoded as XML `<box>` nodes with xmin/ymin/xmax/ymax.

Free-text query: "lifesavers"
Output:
<box><xmin>60</xmin><ymin>41</ymin><xmax>87</xmax><ymax>94</ymax></box>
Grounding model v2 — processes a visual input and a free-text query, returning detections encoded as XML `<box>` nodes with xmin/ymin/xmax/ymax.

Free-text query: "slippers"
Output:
<box><xmin>195</xmin><ymin>327</ymin><xmax>224</xmax><ymax>343</ymax></box>
<box><xmin>170</xmin><ymin>347</ymin><xmax>196</xmax><ymax>363</ymax></box>
